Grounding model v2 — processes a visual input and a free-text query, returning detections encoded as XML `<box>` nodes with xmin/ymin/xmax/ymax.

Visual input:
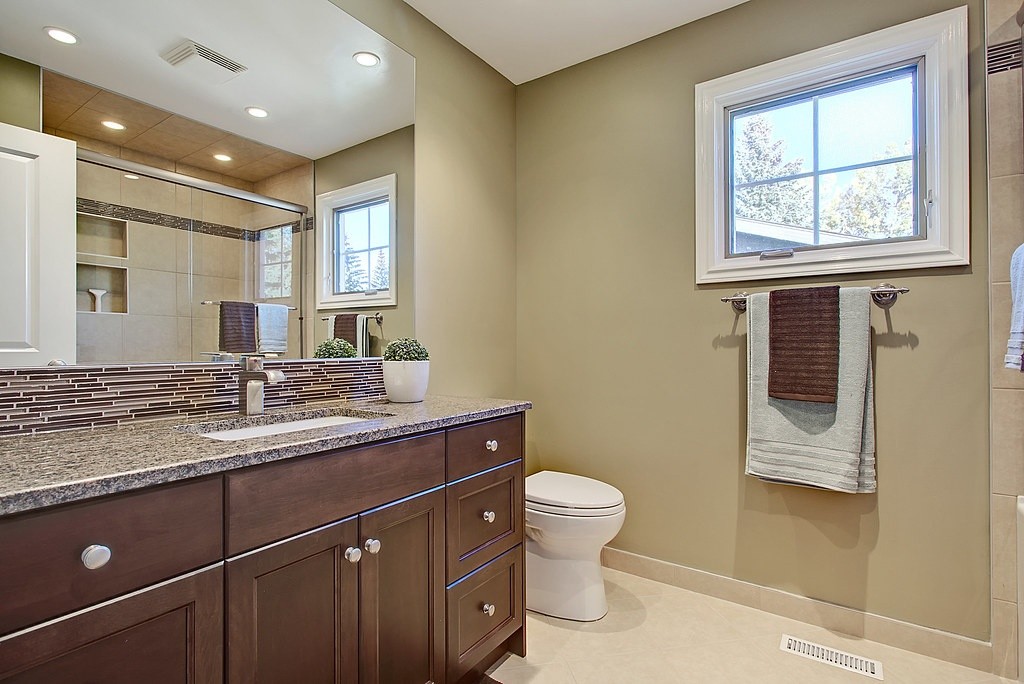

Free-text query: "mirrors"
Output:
<box><xmin>0</xmin><ymin>0</ymin><xmax>419</xmax><ymax>373</ymax></box>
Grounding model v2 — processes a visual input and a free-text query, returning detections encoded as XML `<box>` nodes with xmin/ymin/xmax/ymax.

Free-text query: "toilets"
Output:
<box><xmin>525</xmin><ymin>469</ymin><xmax>627</xmax><ymax>622</ymax></box>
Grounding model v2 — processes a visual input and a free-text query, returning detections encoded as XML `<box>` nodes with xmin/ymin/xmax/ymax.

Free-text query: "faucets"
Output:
<box><xmin>200</xmin><ymin>352</ymin><xmax>235</xmax><ymax>361</ymax></box>
<box><xmin>238</xmin><ymin>353</ymin><xmax>287</xmax><ymax>415</ymax></box>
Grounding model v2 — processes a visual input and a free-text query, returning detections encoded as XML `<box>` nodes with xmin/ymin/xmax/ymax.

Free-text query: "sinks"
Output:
<box><xmin>174</xmin><ymin>407</ymin><xmax>398</xmax><ymax>441</ymax></box>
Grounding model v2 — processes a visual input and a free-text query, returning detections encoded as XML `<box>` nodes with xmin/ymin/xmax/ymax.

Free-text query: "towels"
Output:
<box><xmin>1003</xmin><ymin>243</ymin><xmax>1024</xmax><ymax>371</ymax></box>
<box><xmin>327</xmin><ymin>313</ymin><xmax>369</xmax><ymax>357</ymax></box>
<box><xmin>743</xmin><ymin>285</ymin><xmax>877</xmax><ymax>495</ymax></box>
<box><xmin>219</xmin><ymin>300</ymin><xmax>289</xmax><ymax>354</ymax></box>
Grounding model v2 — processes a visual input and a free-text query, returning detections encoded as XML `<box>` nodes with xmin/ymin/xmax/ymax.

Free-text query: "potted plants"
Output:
<box><xmin>381</xmin><ymin>336</ymin><xmax>429</xmax><ymax>404</ymax></box>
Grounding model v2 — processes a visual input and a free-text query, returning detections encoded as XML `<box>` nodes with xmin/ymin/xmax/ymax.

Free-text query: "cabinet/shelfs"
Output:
<box><xmin>0</xmin><ymin>412</ymin><xmax>528</xmax><ymax>684</ymax></box>
